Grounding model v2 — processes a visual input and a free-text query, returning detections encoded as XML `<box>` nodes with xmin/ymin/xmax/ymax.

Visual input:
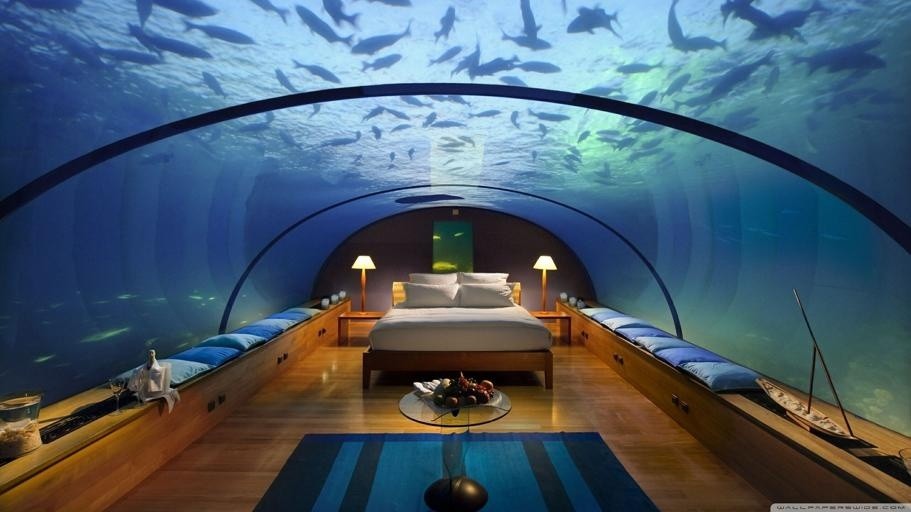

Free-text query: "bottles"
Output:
<box><xmin>144</xmin><ymin>350</ymin><xmax>164</xmax><ymax>393</ymax></box>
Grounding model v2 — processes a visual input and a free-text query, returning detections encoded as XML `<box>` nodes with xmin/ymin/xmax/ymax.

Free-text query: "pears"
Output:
<box><xmin>442</xmin><ymin>378</ymin><xmax>451</xmax><ymax>390</ymax></box>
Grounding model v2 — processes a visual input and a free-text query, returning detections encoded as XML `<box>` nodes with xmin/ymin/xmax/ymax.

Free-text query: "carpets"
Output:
<box><xmin>245</xmin><ymin>432</ymin><xmax>663</xmax><ymax>512</ymax></box>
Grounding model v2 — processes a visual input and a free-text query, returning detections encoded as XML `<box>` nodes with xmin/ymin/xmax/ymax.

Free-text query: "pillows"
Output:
<box><xmin>114</xmin><ymin>305</ymin><xmax>322</xmax><ymax>388</ymax></box>
<box><xmin>458</xmin><ymin>283</ymin><xmax>516</xmax><ymax>307</ymax></box>
<box><xmin>578</xmin><ymin>307</ymin><xmax>766</xmax><ymax>393</ymax></box>
<box><xmin>400</xmin><ymin>281</ymin><xmax>459</xmax><ymax>307</ymax></box>
<box><xmin>407</xmin><ymin>271</ymin><xmax>457</xmax><ymax>285</ymax></box>
<box><xmin>456</xmin><ymin>272</ymin><xmax>509</xmax><ymax>285</ymax></box>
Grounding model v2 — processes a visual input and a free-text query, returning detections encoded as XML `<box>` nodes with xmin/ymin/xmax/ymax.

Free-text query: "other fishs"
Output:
<box><xmin>0</xmin><ymin>0</ymin><xmax>911</xmax><ymax>187</ymax></box>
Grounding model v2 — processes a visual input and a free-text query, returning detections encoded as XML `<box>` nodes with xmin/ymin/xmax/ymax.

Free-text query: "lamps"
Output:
<box><xmin>352</xmin><ymin>255</ymin><xmax>375</xmax><ymax>312</ymax></box>
<box><xmin>533</xmin><ymin>255</ymin><xmax>556</xmax><ymax>315</ymax></box>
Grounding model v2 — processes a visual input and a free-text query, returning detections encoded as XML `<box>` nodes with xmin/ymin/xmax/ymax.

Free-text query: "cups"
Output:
<box><xmin>322</xmin><ymin>290</ymin><xmax>347</xmax><ymax>309</ymax></box>
<box><xmin>560</xmin><ymin>291</ymin><xmax>585</xmax><ymax>312</ymax></box>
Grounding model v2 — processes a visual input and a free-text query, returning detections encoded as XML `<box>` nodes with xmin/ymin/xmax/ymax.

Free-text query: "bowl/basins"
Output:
<box><xmin>136</xmin><ymin>361</ymin><xmax>172</xmax><ymax>395</ymax></box>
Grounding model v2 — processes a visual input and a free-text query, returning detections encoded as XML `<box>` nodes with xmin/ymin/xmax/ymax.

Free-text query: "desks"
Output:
<box><xmin>531</xmin><ymin>312</ymin><xmax>571</xmax><ymax>345</ymax></box>
<box><xmin>396</xmin><ymin>387</ymin><xmax>512</xmax><ymax>511</ymax></box>
<box><xmin>335</xmin><ymin>310</ymin><xmax>384</xmax><ymax>344</ymax></box>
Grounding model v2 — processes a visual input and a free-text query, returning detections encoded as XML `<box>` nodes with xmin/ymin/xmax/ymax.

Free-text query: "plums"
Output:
<box><xmin>445</xmin><ymin>396</ymin><xmax>458</xmax><ymax>408</ymax></box>
<box><xmin>466</xmin><ymin>395</ymin><xmax>477</xmax><ymax>404</ymax></box>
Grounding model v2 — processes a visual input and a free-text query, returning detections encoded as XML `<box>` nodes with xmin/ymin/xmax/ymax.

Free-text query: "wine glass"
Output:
<box><xmin>129</xmin><ymin>367</ymin><xmax>146</xmax><ymax>408</ymax></box>
<box><xmin>108</xmin><ymin>373</ymin><xmax>127</xmax><ymax>414</ymax></box>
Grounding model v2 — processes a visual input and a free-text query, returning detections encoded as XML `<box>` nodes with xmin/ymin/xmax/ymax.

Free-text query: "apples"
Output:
<box><xmin>480</xmin><ymin>379</ymin><xmax>494</xmax><ymax>393</ymax></box>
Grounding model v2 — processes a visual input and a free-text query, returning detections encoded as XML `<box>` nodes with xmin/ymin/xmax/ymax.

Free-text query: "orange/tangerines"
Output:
<box><xmin>434</xmin><ymin>395</ymin><xmax>445</xmax><ymax>405</ymax></box>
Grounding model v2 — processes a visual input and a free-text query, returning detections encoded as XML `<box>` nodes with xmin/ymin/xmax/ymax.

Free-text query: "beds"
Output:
<box><xmin>359</xmin><ymin>271</ymin><xmax>556</xmax><ymax>391</ymax></box>
